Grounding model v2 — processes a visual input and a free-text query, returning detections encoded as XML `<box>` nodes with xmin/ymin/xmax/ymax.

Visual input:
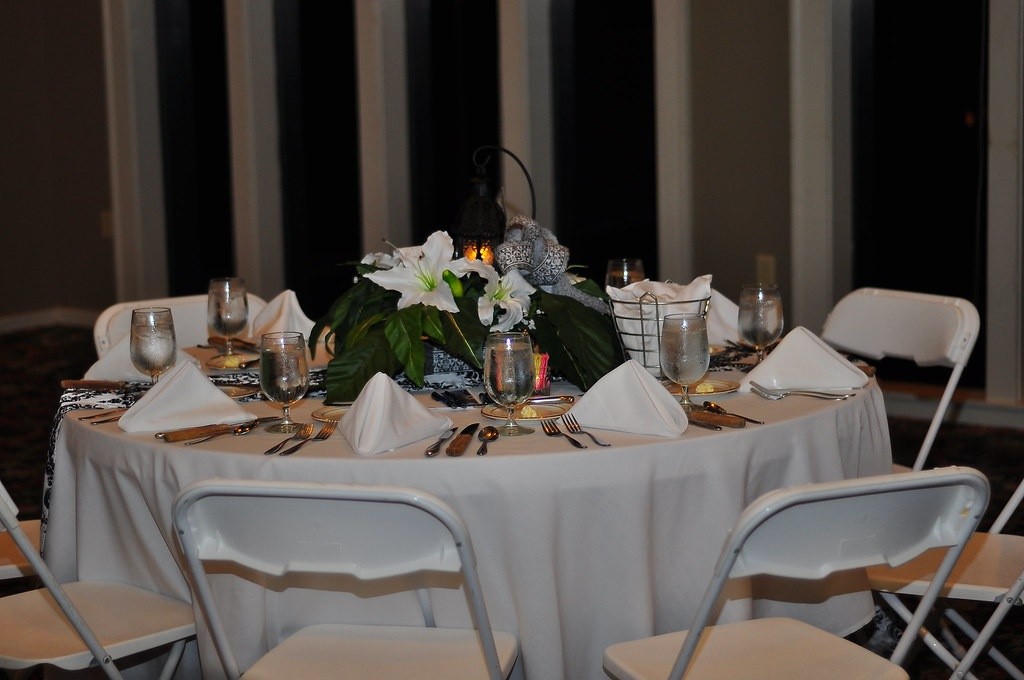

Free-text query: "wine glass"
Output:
<box><xmin>205</xmin><ymin>278</ymin><xmax>249</xmax><ymax>369</ymax></box>
<box><xmin>484</xmin><ymin>331</ymin><xmax>535</xmax><ymax>436</ymax></box>
<box><xmin>737</xmin><ymin>283</ymin><xmax>785</xmax><ymax>373</ymax></box>
<box><xmin>130</xmin><ymin>307</ymin><xmax>177</xmax><ymax>390</ymax></box>
<box><xmin>660</xmin><ymin>314</ymin><xmax>711</xmax><ymax>414</ymax></box>
<box><xmin>260</xmin><ymin>331</ymin><xmax>309</xmax><ymax>433</ymax></box>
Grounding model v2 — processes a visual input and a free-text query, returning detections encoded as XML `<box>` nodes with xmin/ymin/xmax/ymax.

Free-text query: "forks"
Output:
<box><xmin>749</xmin><ymin>380</ymin><xmax>857</xmax><ymax>401</ymax></box>
<box><xmin>264</xmin><ymin>424</ymin><xmax>315</xmax><ymax>456</ymax></box>
<box><xmin>279</xmin><ymin>421</ymin><xmax>340</xmax><ymax>456</ymax></box>
<box><xmin>559</xmin><ymin>412</ymin><xmax>613</xmax><ymax>446</ymax></box>
<box><xmin>540</xmin><ymin>418</ymin><xmax>588</xmax><ymax>448</ymax></box>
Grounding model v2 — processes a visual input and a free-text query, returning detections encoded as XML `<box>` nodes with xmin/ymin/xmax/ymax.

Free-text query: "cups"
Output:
<box><xmin>530</xmin><ymin>366</ymin><xmax>551</xmax><ymax>397</ymax></box>
<box><xmin>604</xmin><ymin>257</ymin><xmax>645</xmax><ymax>296</ymax></box>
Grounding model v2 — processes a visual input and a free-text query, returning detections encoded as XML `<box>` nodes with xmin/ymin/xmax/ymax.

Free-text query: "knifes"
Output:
<box><xmin>446</xmin><ymin>422</ymin><xmax>480</xmax><ymax>456</ymax></box>
<box><xmin>425</xmin><ymin>427</ymin><xmax>459</xmax><ymax>457</ymax></box>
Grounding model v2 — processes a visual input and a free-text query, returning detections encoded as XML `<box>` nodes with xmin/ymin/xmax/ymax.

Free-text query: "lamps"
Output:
<box><xmin>457</xmin><ymin>144</ymin><xmax>538</xmax><ymax>268</ymax></box>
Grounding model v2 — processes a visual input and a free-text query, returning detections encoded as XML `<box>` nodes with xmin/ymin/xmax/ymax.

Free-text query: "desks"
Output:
<box><xmin>40</xmin><ymin>340</ymin><xmax>893</xmax><ymax>680</ymax></box>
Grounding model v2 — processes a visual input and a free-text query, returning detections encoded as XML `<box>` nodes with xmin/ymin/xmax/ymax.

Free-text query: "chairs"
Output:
<box><xmin>821</xmin><ymin>287</ymin><xmax>980</xmax><ymax>478</ymax></box>
<box><xmin>0</xmin><ymin>481</ymin><xmax>197</xmax><ymax>680</ymax></box>
<box><xmin>863</xmin><ymin>479</ymin><xmax>1024</xmax><ymax>680</ymax></box>
<box><xmin>170</xmin><ymin>478</ymin><xmax>525</xmax><ymax>680</ymax></box>
<box><xmin>94</xmin><ymin>292</ymin><xmax>269</xmax><ymax>363</ymax></box>
<box><xmin>602</xmin><ymin>465</ymin><xmax>993</xmax><ymax>680</ymax></box>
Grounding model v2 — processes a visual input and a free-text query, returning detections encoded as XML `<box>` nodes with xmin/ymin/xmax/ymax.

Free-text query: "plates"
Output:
<box><xmin>480</xmin><ymin>403</ymin><xmax>573</xmax><ymax>420</ymax></box>
<box><xmin>312</xmin><ymin>404</ymin><xmax>351</xmax><ymax>424</ymax></box>
<box><xmin>660</xmin><ymin>377</ymin><xmax>741</xmax><ymax>397</ymax></box>
<box><xmin>214</xmin><ymin>384</ymin><xmax>261</xmax><ymax>402</ymax></box>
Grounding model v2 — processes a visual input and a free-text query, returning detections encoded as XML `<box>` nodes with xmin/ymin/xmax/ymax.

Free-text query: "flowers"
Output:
<box><xmin>309</xmin><ymin>226</ymin><xmax>639</xmax><ymax>404</ymax></box>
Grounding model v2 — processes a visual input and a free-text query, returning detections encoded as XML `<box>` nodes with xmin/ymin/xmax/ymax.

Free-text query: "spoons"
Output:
<box><xmin>476</xmin><ymin>426</ymin><xmax>499</xmax><ymax>455</ymax></box>
<box><xmin>184</xmin><ymin>419</ymin><xmax>259</xmax><ymax>445</ymax></box>
<box><xmin>703</xmin><ymin>400</ymin><xmax>762</xmax><ymax>424</ymax></box>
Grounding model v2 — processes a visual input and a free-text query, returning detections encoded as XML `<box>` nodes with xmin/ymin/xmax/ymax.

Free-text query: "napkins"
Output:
<box><xmin>601</xmin><ymin>274</ymin><xmax>756</xmax><ymax>380</ymax></box>
<box><xmin>232</xmin><ymin>288</ymin><xmax>339</xmax><ymax>346</ymax></box>
<box><xmin>336</xmin><ymin>372</ymin><xmax>454</xmax><ymax>457</ymax></box>
<box><xmin>82</xmin><ymin>333</ymin><xmax>202</xmax><ymax>384</ymax></box>
<box><xmin>559</xmin><ymin>359</ymin><xmax>686</xmax><ymax>437</ymax></box>
<box><xmin>737</xmin><ymin>326</ymin><xmax>869</xmax><ymax>388</ymax></box>
<box><xmin>117</xmin><ymin>357</ymin><xmax>259</xmax><ymax>434</ymax></box>
